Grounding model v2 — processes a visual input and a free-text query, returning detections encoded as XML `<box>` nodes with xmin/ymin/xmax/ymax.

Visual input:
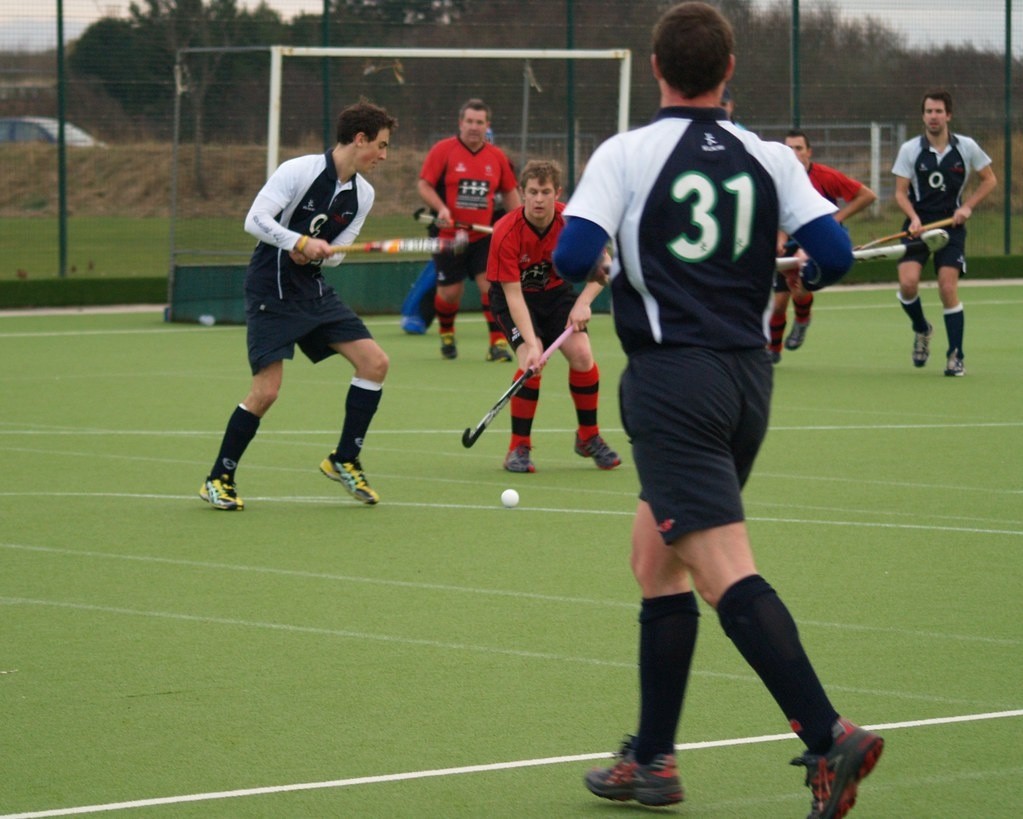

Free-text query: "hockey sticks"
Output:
<box><xmin>332</xmin><ymin>229</ymin><xmax>470</xmax><ymax>255</ymax></box>
<box><xmin>460</xmin><ymin>323</ymin><xmax>574</xmax><ymax>450</ymax></box>
<box><xmin>413</xmin><ymin>207</ymin><xmax>493</xmax><ymax>234</ymax></box>
<box><xmin>774</xmin><ymin>227</ymin><xmax>951</xmax><ymax>272</ymax></box>
<box><xmin>850</xmin><ymin>214</ymin><xmax>954</xmax><ymax>251</ymax></box>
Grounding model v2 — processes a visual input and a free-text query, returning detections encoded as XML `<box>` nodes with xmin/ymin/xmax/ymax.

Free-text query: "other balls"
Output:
<box><xmin>501</xmin><ymin>488</ymin><xmax>520</xmax><ymax>507</ymax></box>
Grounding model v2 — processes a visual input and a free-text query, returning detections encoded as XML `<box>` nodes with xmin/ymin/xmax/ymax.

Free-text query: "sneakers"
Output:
<box><xmin>785</xmin><ymin>318</ymin><xmax>811</xmax><ymax>349</ymax></box>
<box><xmin>505</xmin><ymin>442</ymin><xmax>535</xmax><ymax>473</ymax></box>
<box><xmin>765</xmin><ymin>349</ymin><xmax>780</xmax><ymax>363</ymax></box>
<box><xmin>912</xmin><ymin>323</ymin><xmax>933</xmax><ymax>367</ymax></box>
<box><xmin>440</xmin><ymin>332</ymin><xmax>458</xmax><ymax>359</ymax></box>
<box><xmin>485</xmin><ymin>340</ymin><xmax>514</xmax><ymax>362</ymax></box>
<box><xmin>199</xmin><ymin>475</ymin><xmax>245</xmax><ymax>511</ymax></box>
<box><xmin>787</xmin><ymin>717</ymin><xmax>881</xmax><ymax>819</ymax></box>
<box><xmin>945</xmin><ymin>346</ymin><xmax>965</xmax><ymax>377</ymax></box>
<box><xmin>319</xmin><ymin>450</ymin><xmax>379</xmax><ymax>504</ymax></box>
<box><xmin>585</xmin><ymin>734</ymin><xmax>686</xmax><ymax>807</ymax></box>
<box><xmin>573</xmin><ymin>429</ymin><xmax>622</xmax><ymax>471</ymax></box>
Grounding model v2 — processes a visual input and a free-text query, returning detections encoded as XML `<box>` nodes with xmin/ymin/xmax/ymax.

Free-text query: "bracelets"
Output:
<box><xmin>296</xmin><ymin>234</ymin><xmax>309</xmax><ymax>252</ymax></box>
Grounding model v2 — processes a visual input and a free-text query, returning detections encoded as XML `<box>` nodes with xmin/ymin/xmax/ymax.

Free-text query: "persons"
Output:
<box><xmin>417</xmin><ymin>99</ymin><xmax>522</xmax><ymax>362</ymax></box>
<box><xmin>552</xmin><ymin>0</ymin><xmax>890</xmax><ymax>818</ymax></box>
<box><xmin>198</xmin><ymin>102</ymin><xmax>390</xmax><ymax>511</ymax></box>
<box><xmin>485</xmin><ymin>158</ymin><xmax>624</xmax><ymax>474</ymax></box>
<box><xmin>718</xmin><ymin>87</ymin><xmax>734</xmax><ymax>119</ymax></box>
<box><xmin>767</xmin><ymin>129</ymin><xmax>878</xmax><ymax>363</ymax></box>
<box><xmin>886</xmin><ymin>91</ymin><xmax>997</xmax><ymax>376</ymax></box>
